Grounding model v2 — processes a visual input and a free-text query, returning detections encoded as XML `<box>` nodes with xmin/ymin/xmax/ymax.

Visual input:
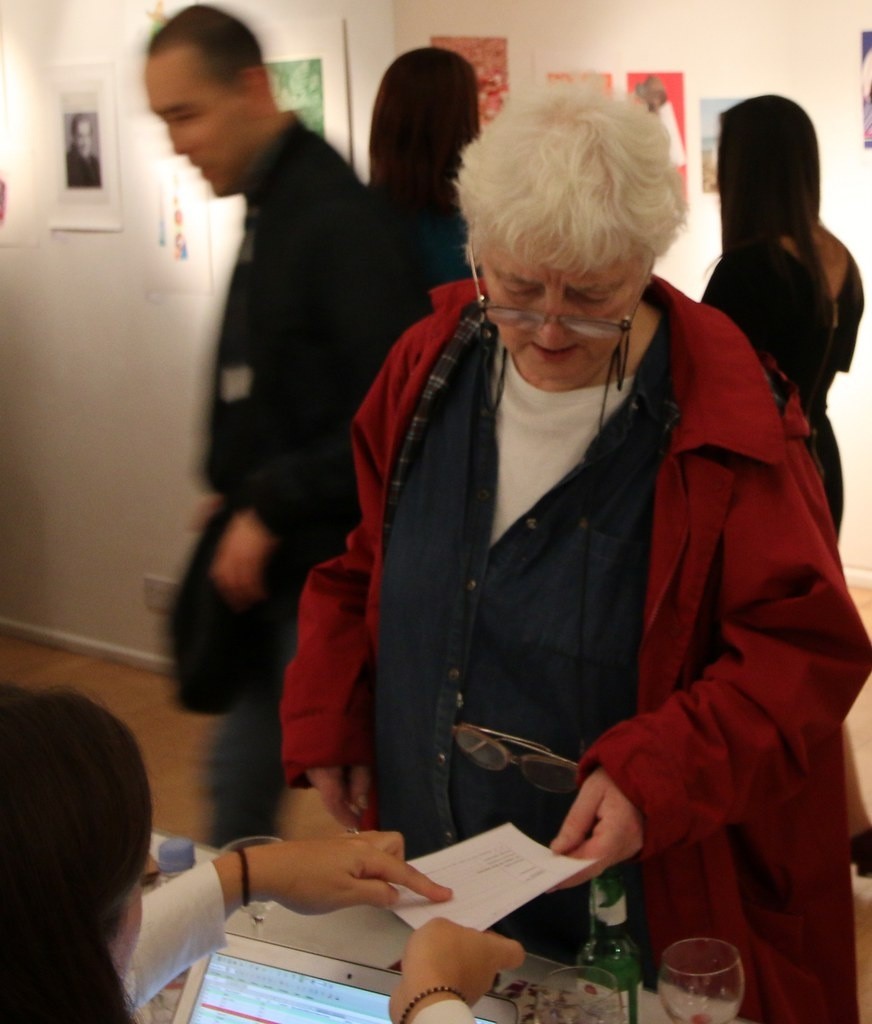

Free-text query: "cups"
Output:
<box><xmin>532</xmin><ymin>965</ymin><xmax>625</xmax><ymax>1024</ymax></box>
<box><xmin>215</xmin><ymin>835</ymin><xmax>282</xmax><ymax>858</ymax></box>
<box><xmin>656</xmin><ymin>937</ymin><xmax>745</xmax><ymax>1023</ymax></box>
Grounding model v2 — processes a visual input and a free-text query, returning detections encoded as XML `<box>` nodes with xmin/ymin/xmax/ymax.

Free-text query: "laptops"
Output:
<box><xmin>171</xmin><ymin>932</ymin><xmax>520</xmax><ymax>1024</ymax></box>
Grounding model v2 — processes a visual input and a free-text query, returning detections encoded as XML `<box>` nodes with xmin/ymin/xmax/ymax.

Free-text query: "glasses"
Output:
<box><xmin>449</xmin><ymin>722</ymin><xmax>578</xmax><ymax>793</ymax></box>
<box><xmin>470</xmin><ymin>242</ymin><xmax>653</xmax><ymax>337</ymax></box>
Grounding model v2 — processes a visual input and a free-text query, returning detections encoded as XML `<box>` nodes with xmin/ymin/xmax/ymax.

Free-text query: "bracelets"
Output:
<box><xmin>399</xmin><ymin>986</ymin><xmax>470</xmax><ymax>1024</ymax></box>
<box><xmin>235</xmin><ymin>846</ymin><xmax>250</xmax><ymax>906</ymax></box>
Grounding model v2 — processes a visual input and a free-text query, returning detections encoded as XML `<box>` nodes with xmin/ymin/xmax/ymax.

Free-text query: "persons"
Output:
<box><xmin>67</xmin><ymin>113</ymin><xmax>101</xmax><ymax>189</ymax></box>
<box><xmin>632</xmin><ymin>75</ymin><xmax>687</xmax><ymax>196</ymax></box>
<box><xmin>700</xmin><ymin>94</ymin><xmax>872</xmax><ymax>880</ymax></box>
<box><xmin>0</xmin><ymin>681</ymin><xmax>525</xmax><ymax>1024</ymax></box>
<box><xmin>280</xmin><ymin>84</ymin><xmax>872</xmax><ymax>1024</ymax></box>
<box><xmin>143</xmin><ymin>5</ymin><xmax>479</xmax><ymax>849</ymax></box>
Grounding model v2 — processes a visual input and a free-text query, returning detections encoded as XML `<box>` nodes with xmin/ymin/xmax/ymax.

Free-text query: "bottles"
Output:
<box><xmin>155</xmin><ymin>837</ymin><xmax>195</xmax><ymax>887</ymax></box>
<box><xmin>575</xmin><ymin>869</ymin><xmax>641</xmax><ymax>1024</ymax></box>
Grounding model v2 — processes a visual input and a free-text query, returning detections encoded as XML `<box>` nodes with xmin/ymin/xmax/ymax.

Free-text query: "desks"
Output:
<box><xmin>128</xmin><ymin>834</ymin><xmax>750</xmax><ymax>1024</ymax></box>
